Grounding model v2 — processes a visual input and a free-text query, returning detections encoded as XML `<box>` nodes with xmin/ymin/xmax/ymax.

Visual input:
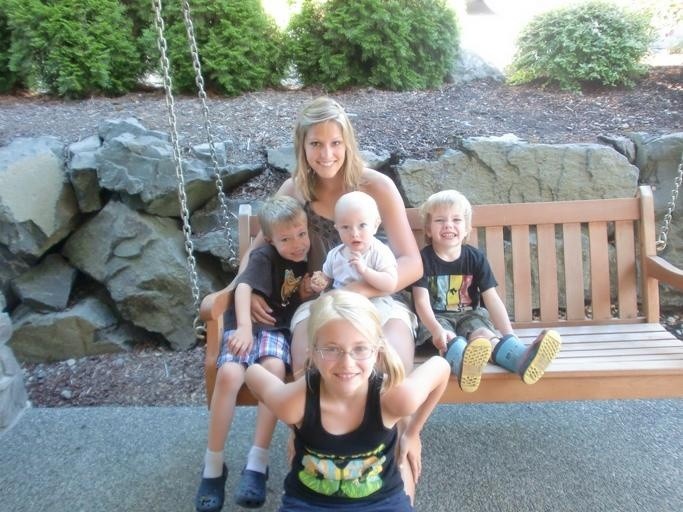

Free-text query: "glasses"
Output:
<box><xmin>309</xmin><ymin>342</ymin><xmax>377</xmax><ymax>362</ymax></box>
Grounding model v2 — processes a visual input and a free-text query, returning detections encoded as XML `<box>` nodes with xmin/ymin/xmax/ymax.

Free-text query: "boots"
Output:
<box><xmin>488</xmin><ymin>325</ymin><xmax>562</xmax><ymax>387</ymax></box>
<box><xmin>440</xmin><ymin>330</ymin><xmax>493</xmax><ymax>395</ymax></box>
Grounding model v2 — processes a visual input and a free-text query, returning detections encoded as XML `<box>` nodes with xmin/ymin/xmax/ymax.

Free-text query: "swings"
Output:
<box><xmin>153</xmin><ymin>1</ymin><xmax>683</xmax><ymax>410</ymax></box>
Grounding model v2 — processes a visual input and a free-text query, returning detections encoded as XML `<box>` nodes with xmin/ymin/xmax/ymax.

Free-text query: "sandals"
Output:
<box><xmin>193</xmin><ymin>462</ymin><xmax>229</xmax><ymax>512</ymax></box>
<box><xmin>232</xmin><ymin>456</ymin><xmax>271</xmax><ymax>508</ymax></box>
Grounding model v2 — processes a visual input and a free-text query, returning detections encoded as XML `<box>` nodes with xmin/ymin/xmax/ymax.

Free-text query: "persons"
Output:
<box><xmin>402</xmin><ymin>187</ymin><xmax>563</xmax><ymax>393</ymax></box>
<box><xmin>242</xmin><ymin>288</ymin><xmax>452</xmax><ymax>511</ymax></box>
<box><xmin>309</xmin><ymin>190</ymin><xmax>398</xmax><ymax>332</ymax></box>
<box><xmin>235</xmin><ymin>97</ymin><xmax>425</xmax><ymax>507</ymax></box>
<box><xmin>192</xmin><ymin>193</ymin><xmax>313</xmax><ymax>511</ymax></box>
